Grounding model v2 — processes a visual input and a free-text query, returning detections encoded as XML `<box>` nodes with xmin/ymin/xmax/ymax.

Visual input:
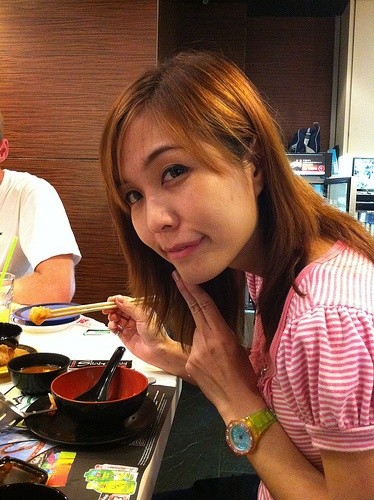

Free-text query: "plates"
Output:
<box><xmin>13</xmin><ymin>314</ymin><xmax>82</xmax><ymax>332</ymax></box>
<box><xmin>0</xmin><ymin>344</ymin><xmax>36</xmax><ymax>374</ymax></box>
<box><xmin>24</xmin><ymin>397</ymin><xmax>156</xmax><ymax>445</ymax></box>
<box><xmin>14</xmin><ymin>303</ymin><xmax>81</xmax><ymax>321</ymax></box>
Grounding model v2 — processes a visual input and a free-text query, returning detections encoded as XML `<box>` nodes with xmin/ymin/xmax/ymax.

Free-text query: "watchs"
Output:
<box><xmin>225</xmin><ymin>406</ymin><xmax>276</xmax><ymax>457</ymax></box>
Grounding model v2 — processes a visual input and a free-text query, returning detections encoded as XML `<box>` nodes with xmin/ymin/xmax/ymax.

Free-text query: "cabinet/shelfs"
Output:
<box><xmin>0</xmin><ymin>0</ymin><xmax>159</xmax><ymax>306</ymax></box>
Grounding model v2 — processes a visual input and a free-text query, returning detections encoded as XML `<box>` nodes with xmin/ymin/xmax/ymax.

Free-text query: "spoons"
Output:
<box><xmin>73</xmin><ymin>347</ymin><xmax>125</xmax><ymax>402</ymax></box>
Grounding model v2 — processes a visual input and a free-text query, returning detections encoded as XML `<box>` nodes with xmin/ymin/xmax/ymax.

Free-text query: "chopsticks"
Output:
<box><xmin>51</xmin><ymin>297</ymin><xmax>156</xmax><ymax>317</ymax></box>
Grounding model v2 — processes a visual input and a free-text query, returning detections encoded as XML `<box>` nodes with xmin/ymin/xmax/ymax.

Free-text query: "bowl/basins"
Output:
<box><xmin>7</xmin><ymin>353</ymin><xmax>70</xmax><ymax>394</ymax></box>
<box><xmin>0</xmin><ymin>322</ymin><xmax>22</xmax><ymax>341</ymax></box>
<box><xmin>51</xmin><ymin>366</ymin><xmax>148</xmax><ymax>422</ymax></box>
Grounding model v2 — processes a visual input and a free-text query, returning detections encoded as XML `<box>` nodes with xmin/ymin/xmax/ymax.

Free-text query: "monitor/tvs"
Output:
<box><xmin>323</xmin><ymin>176</ymin><xmax>357</xmax><ymax>219</ymax></box>
<box><xmin>352</xmin><ymin>156</ymin><xmax>374</xmax><ymax>192</ymax></box>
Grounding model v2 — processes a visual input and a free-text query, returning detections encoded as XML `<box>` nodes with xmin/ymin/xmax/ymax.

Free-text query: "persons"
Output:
<box><xmin>99</xmin><ymin>49</ymin><xmax>374</xmax><ymax>500</ymax></box>
<box><xmin>0</xmin><ymin>112</ymin><xmax>82</xmax><ymax>307</ymax></box>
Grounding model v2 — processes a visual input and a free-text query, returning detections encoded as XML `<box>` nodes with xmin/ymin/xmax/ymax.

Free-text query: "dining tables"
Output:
<box><xmin>0</xmin><ymin>305</ymin><xmax>183</xmax><ymax>500</ymax></box>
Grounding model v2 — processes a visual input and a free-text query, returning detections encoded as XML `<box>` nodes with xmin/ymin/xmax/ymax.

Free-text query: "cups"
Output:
<box><xmin>0</xmin><ymin>483</ymin><xmax>68</xmax><ymax>500</ymax></box>
<box><xmin>0</xmin><ymin>273</ymin><xmax>15</xmax><ymax>322</ymax></box>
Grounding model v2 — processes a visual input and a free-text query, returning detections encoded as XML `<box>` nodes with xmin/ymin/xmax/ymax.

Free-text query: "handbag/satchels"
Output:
<box><xmin>288</xmin><ymin>121</ymin><xmax>321</xmax><ymax>154</ymax></box>
